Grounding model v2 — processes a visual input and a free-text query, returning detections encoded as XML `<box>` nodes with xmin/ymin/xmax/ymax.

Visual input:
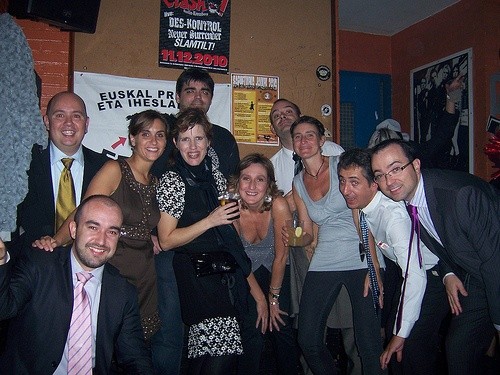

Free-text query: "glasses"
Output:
<box><xmin>373</xmin><ymin>162</ymin><xmax>412</xmax><ymax>184</ymax></box>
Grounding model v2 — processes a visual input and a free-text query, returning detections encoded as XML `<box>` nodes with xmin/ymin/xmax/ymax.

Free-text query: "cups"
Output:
<box><xmin>284</xmin><ymin>219</ymin><xmax>304</xmax><ymax>247</ymax></box>
<box><xmin>220</xmin><ymin>198</ymin><xmax>240</xmax><ymax>221</ymax></box>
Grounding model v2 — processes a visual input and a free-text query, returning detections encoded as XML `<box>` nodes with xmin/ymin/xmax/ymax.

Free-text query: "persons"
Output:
<box><xmin>417</xmin><ymin>63</ymin><xmax>461</xmax><ymax>163</ymax></box>
<box><xmin>0</xmin><ymin>67</ymin><xmax>500</xmax><ymax>375</ymax></box>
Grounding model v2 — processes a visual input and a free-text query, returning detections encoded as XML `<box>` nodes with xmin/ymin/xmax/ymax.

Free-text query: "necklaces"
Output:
<box><xmin>305</xmin><ymin>156</ymin><xmax>324</xmax><ymax>180</ymax></box>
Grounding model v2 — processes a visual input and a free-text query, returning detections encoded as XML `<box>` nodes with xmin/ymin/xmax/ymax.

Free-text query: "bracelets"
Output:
<box><xmin>270</xmin><ymin>285</ymin><xmax>282</xmax><ymax>290</ymax></box>
<box><xmin>269</xmin><ymin>301</ymin><xmax>279</xmax><ymax>305</ymax></box>
<box><xmin>269</xmin><ymin>290</ymin><xmax>280</xmax><ymax>299</ymax></box>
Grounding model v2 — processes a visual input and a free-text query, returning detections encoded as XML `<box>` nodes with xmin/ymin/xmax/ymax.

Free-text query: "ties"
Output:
<box><xmin>293</xmin><ymin>156</ymin><xmax>303</xmax><ymax>176</ymax></box>
<box><xmin>56</xmin><ymin>157</ymin><xmax>77</xmax><ymax>248</ymax></box>
<box><xmin>67</xmin><ymin>272</ymin><xmax>94</xmax><ymax>375</ymax></box>
<box><xmin>360</xmin><ymin>215</ymin><xmax>381</xmax><ymax>316</ymax></box>
<box><xmin>395</xmin><ymin>206</ymin><xmax>423</xmax><ymax>337</ymax></box>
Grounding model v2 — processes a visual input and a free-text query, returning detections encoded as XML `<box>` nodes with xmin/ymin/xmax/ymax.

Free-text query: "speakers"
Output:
<box><xmin>7</xmin><ymin>0</ymin><xmax>102</xmax><ymax>35</ymax></box>
